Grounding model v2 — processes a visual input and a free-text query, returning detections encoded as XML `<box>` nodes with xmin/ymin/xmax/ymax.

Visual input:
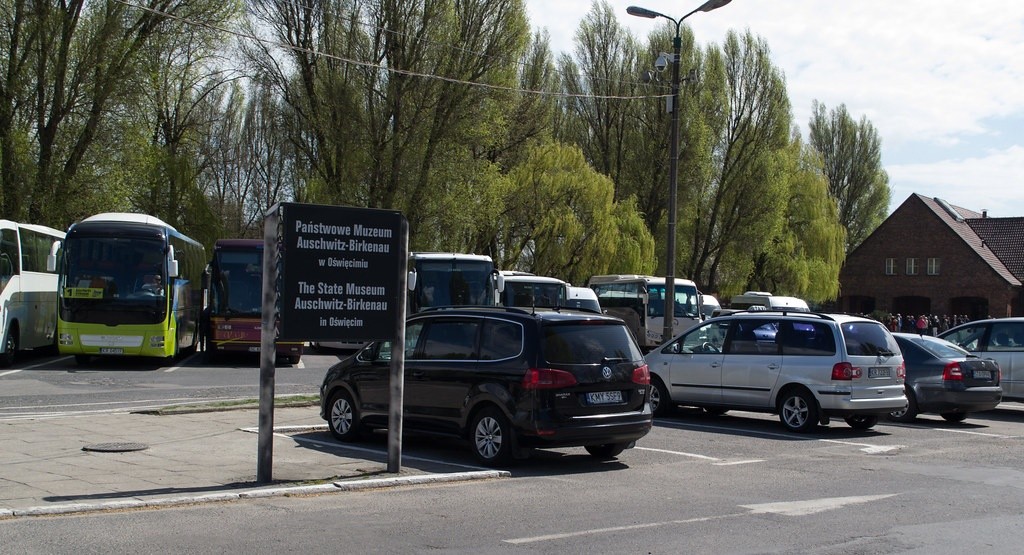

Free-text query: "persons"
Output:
<box><xmin>843</xmin><ymin>312</ymin><xmax>997</xmax><ymax>337</ymax></box>
<box><xmin>142</xmin><ymin>275</ymin><xmax>164</xmax><ymax>296</ymax></box>
<box><xmin>199</xmin><ymin>306</ymin><xmax>209</xmax><ymax>352</ymax></box>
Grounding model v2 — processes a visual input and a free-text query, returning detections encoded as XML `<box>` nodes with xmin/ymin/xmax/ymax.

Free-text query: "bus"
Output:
<box><xmin>0</xmin><ymin>220</ymin><xmax>67</xmax><ymax>369</ymax></box>
<box><xmin>199</xmin><ymin>238</ymin><xmax>304</xmax><ymax>365</ymax></box>
<box><xmin>314</xmin><ymin>251</ymin><xmax>809</xmax><ymax>355</ymax></box>
<box><xmin>47</xmin><ymin>213</ymin><xmax>207</xmax><ymax>366</ymax></box>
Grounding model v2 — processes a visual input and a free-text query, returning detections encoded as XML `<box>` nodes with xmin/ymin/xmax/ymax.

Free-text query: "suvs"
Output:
<box><xmin>320</xmin><ymin>303</ymin><xmax>654</xmax><ymax>470</ymax></box>
<box><xmin>644</xmin><ymin>308</ymin><xmax>907</xmax><ymax>435</ymax></box>
<box><xmin>933</xmin><ymin>317</ymin><xmax>1024</xmax><ymax>401</ymax></box>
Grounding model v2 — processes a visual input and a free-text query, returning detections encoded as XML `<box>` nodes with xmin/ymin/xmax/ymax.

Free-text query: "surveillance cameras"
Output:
<box><xmin>655</xmin><ymin>56</ymin><xmax>667</xmax><ymax>71</ymax></box>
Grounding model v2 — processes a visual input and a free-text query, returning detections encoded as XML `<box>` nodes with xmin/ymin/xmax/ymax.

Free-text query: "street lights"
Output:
<box><xmin>626</xmin><ymin>0</ymin><xmax>733</xmax><ymax>351</ymax></box>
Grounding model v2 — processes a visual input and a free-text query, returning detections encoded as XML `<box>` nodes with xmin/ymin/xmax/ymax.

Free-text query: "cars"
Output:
<box><xmin>706</xmin><ymin>305</ymin><xmax>1002</xmax><ymax>423</ymax></box>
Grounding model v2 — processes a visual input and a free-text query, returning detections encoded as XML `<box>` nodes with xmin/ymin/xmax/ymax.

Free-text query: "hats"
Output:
<box><xmin>897</xmin><ymin>313</ymin><xmax>901</xmax><ymax>316</ymax></box>
<box><xmin>892</xmin><ymin>316</ymin><xmax>895</xmax><ymax>319</ymax></box>
<box><xmin>890</xmin><ymin>313</ymin><xmax>893</xmax><ymax>315</ymax></box>
